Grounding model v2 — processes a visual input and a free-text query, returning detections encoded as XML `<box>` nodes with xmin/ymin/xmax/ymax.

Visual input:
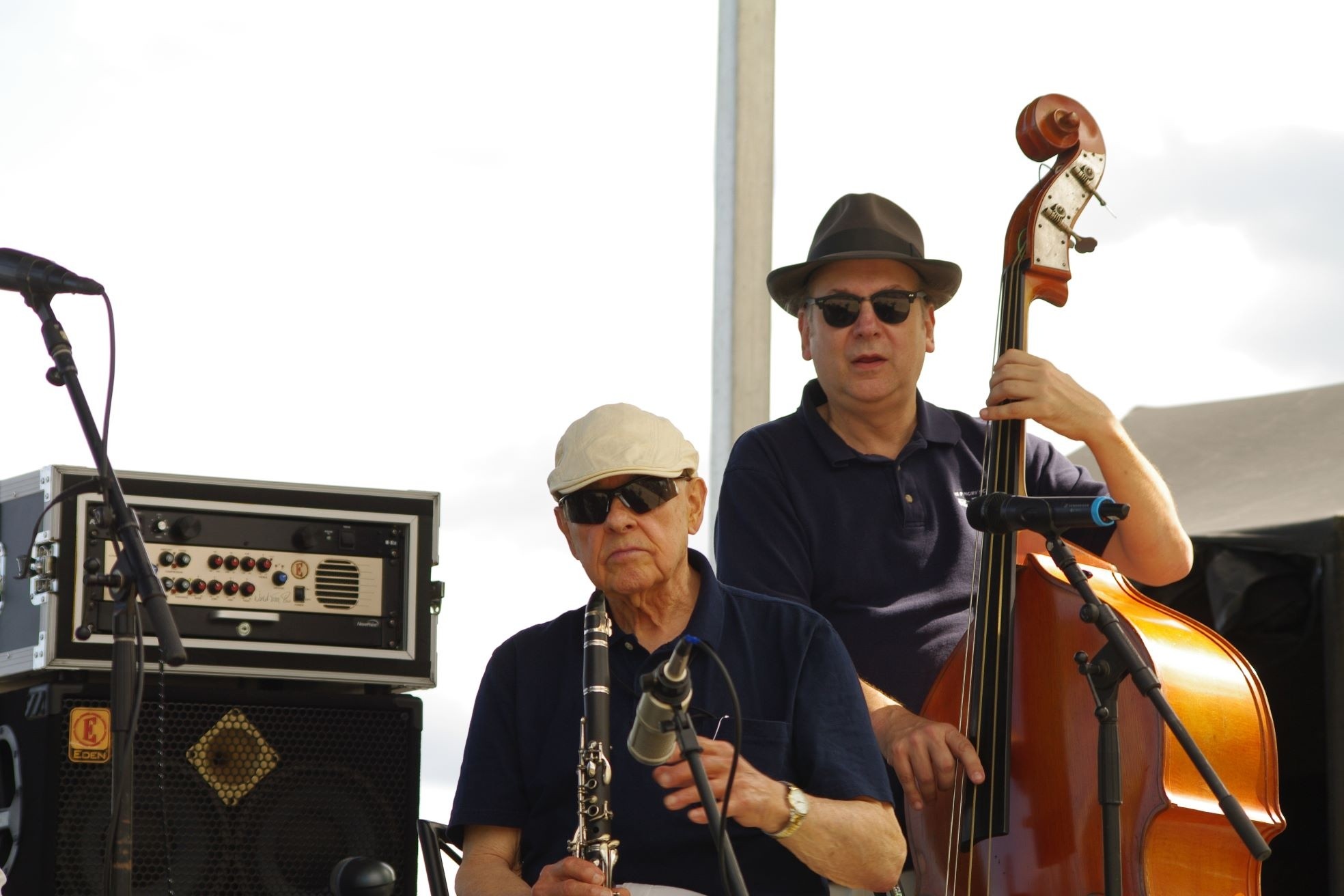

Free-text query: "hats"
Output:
<box><xmin>766</xmin><ymin>192</ymin><xmax>963</xmax><ymax>312</ymax></box>
<box><xmin>546</xmin><ymin>403</ymin><xmax>699</xmax><ymax>504</ymax></box>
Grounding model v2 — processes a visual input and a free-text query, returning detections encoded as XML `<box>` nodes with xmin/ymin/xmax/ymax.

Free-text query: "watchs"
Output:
<box><xmin>766</xmin><ymin>780</ymin><xmax>810</xmax><ymax>839</ymax></box>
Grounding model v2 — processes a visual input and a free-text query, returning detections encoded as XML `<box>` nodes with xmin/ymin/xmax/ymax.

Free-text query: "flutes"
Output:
<box><xmin>575</xmin><ymin>588</ymin><xmax>626</xmax><ymax>889</ymax></box>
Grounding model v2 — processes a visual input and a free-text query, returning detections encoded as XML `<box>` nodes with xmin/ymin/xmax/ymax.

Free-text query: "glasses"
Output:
<box><xmin>558</xmin><ymin>475</ymin><xmax>690</xmax><ymax>525</ymax></box>
<box><xmin>804</xmin><ymin>289</ymin><xmax>927</xmax><ymax>328</ymax></box>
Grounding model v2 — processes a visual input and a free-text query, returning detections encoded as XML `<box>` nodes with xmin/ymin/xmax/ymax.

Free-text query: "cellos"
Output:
<box><xmin>904</xmin><ymin>83</ymin><xmax>1298</xmax><ymax>896</ymax></box>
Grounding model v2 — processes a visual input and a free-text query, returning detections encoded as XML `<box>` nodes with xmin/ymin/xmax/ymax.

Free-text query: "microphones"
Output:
<box><xmin>0</xmin><ymin>247</ymin><xmax>104</xmax><ymax>296</ymax></box>
<box><xmin>328</xmin><ymin>855</ymin><xmax>396</xmax><ymax>896</ymax></box>
<box><xmin>967</xmin><ymin>493</ymin><xmax>1130</xmax><ymax>535</ymax></box>
<box><xmin>625</xmin><ymin>632</ymin><xmax>697</xmax><ymax>766</ymax></box>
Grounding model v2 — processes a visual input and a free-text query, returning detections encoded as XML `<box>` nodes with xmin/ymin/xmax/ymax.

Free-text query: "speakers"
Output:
<box><xmin>1126</xmin><ymin>532</ymin><xmax>1344</xmax><ymax>895</ymax></box>
<box><xmin>1</xmin><ymin>674</ymin><xmax>423</xmax><ymax>896</ymax></box>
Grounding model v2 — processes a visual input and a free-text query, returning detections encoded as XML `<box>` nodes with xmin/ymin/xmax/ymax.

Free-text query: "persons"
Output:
<box><xmin>714</xmin><ymin>192</ymin><xmax>1193</xmax><ymax>896</ymax></box>
<box><xmin>449</xmin><ymin>403</ymin><xmax>908</xmax><ymax>896</ymax></box>
